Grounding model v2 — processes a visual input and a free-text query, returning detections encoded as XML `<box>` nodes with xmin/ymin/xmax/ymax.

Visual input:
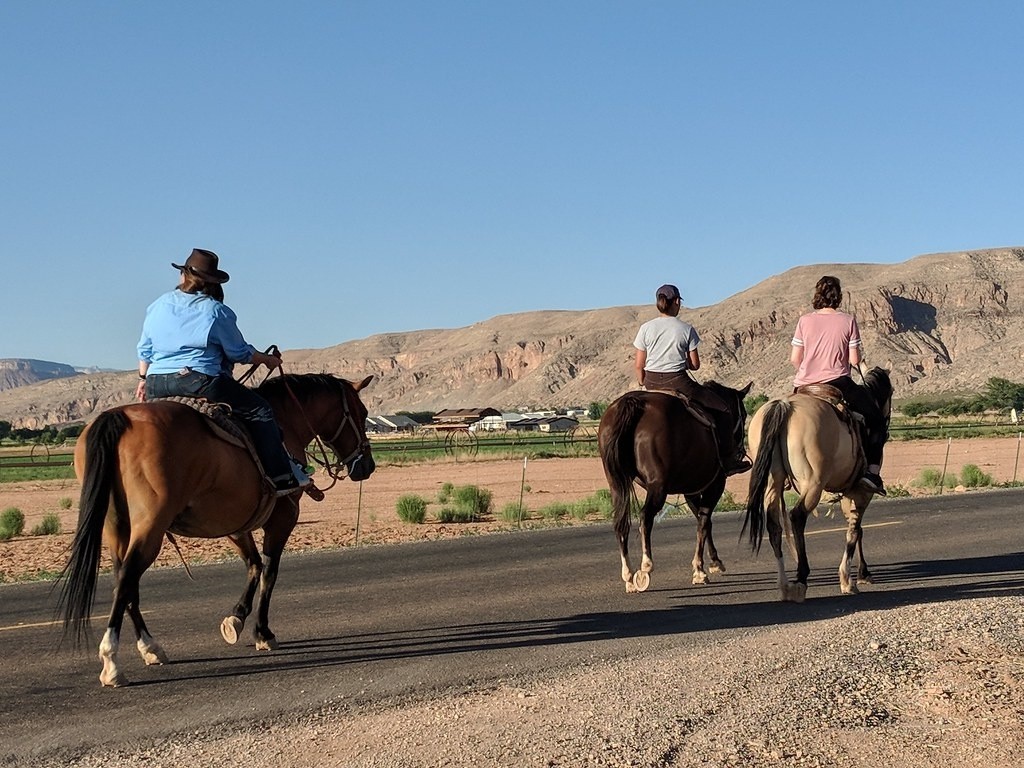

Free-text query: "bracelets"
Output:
<box><xmin>138</xmin><ymin>371</ymin><xmax>146</xmax><ymax>379</ymax></box>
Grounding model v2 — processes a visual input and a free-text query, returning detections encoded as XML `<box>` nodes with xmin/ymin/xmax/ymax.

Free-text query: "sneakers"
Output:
<box><xmin>859</xmin><ymin>470</ymin><xmax>887</xmax><ymax>497</ymax></box>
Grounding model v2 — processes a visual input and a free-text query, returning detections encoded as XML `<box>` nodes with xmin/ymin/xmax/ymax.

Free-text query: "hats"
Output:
<box><xmin>656</xmin><ymin>284</ymin><xmax>684</xmax><ymax>302</ymax></box>
<box><xmin>171</xmin><ymin>248</ymin><xmax>229</xmax><ymax>283</ymax></box>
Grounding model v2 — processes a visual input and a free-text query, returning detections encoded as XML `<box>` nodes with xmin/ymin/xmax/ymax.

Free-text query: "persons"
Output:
<box><xmin>790</xmin><ymin>276</ymin><xmax>887</xmax><ymax>497</ymax></box>
<box><xmin>632</xmin><ymin>285</ymin><xmax>750</xmax><ymax>476</ymax></box>
<box><xmin>136</xmin><ymin>248</ymin><xmax>311</xmax><ymax>497</ymax></box>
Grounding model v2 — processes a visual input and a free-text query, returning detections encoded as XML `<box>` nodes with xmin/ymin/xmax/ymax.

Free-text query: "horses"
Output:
<box><xmin>46</xmin><ymin>373</ymin><xmax>377</xmax><ymax>690</ymax></box>
<box><xmin>737</xmin><ymin>358</ymin><xmax>894</xmax><ymax>603</ymax></box>
<box><xmin>598</xmin><ymin>380</ymin><xmax>754</xmax><ymax>592</ymax></box>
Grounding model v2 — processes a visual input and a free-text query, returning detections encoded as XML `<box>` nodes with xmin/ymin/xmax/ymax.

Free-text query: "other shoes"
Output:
<box><xmin>724</xmin><ymin>460</ymin><xmax>750</xmax><ymax>476</ymax></box>
<box><xmin>274</xmin><ymin>476</ymin><xmax>314</xmax><ymax>497</ymax></box>
